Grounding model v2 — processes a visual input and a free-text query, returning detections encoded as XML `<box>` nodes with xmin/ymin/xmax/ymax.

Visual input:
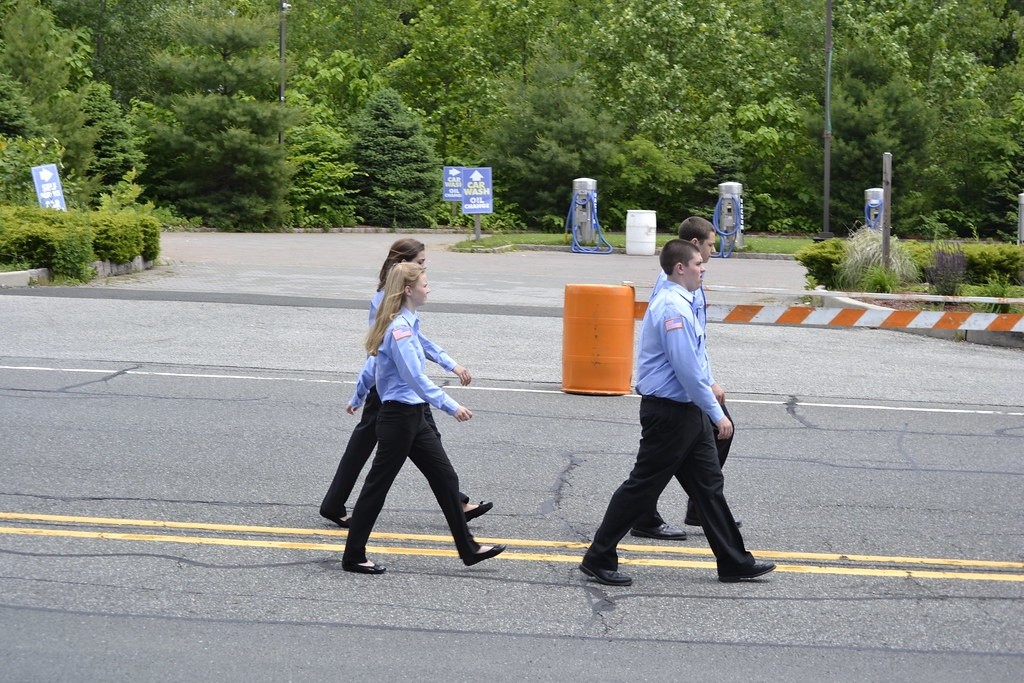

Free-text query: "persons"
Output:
<box><xmin>319</xmin><ymin>237</ymin><xmax>508</xmax><ymax>574</ymax></box>
<box><xmin>578</xmin><ymin>217</ymin><xmax>777</xmax><ymax>587</ymax></box>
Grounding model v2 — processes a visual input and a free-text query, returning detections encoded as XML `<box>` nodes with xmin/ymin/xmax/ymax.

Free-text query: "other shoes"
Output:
<box><xmin>463</xmin><ymin>501</ymin><xmax>494</xmax><ymax>523</ymax></box>
<box><xmin>320</xmin><ymin>508</ymin><xmax>352</xmax><ymax>527</ymax></box>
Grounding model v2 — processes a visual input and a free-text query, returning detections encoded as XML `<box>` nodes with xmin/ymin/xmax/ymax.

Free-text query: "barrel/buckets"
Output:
<box><xmin>561</xmin><ymin>283</ymin><xmax>637</xmax><ymax>397</ymax></box>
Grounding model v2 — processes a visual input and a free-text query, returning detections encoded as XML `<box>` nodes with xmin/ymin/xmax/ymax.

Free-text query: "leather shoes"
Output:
<box><xmin>579</xmin><ymin>563</ymin><xmax>633</xmax><ymax>586</ymax></box>
<box><xmin>343</xmin><ymin>559</ymin><xmax>386</xmax><ymax>574</ymax></box>
<box><xmin>717</xmin><ymin>560</ymin><xmax>777</xmax><ymax>582</ymax></box>
<box><xmin>463</xmin><ymin>544</ymin><xmax>507</xmax><ymax>566</ymax></box>
<box><xmin>684</xmin><ymin>512</ymin><xmax>743</xmax><ymax>528</ymax></box>
<box><xmin>631</xmin><ymin>523</ymin><xmax>686</xmax><ymax>540</ymax></box>
